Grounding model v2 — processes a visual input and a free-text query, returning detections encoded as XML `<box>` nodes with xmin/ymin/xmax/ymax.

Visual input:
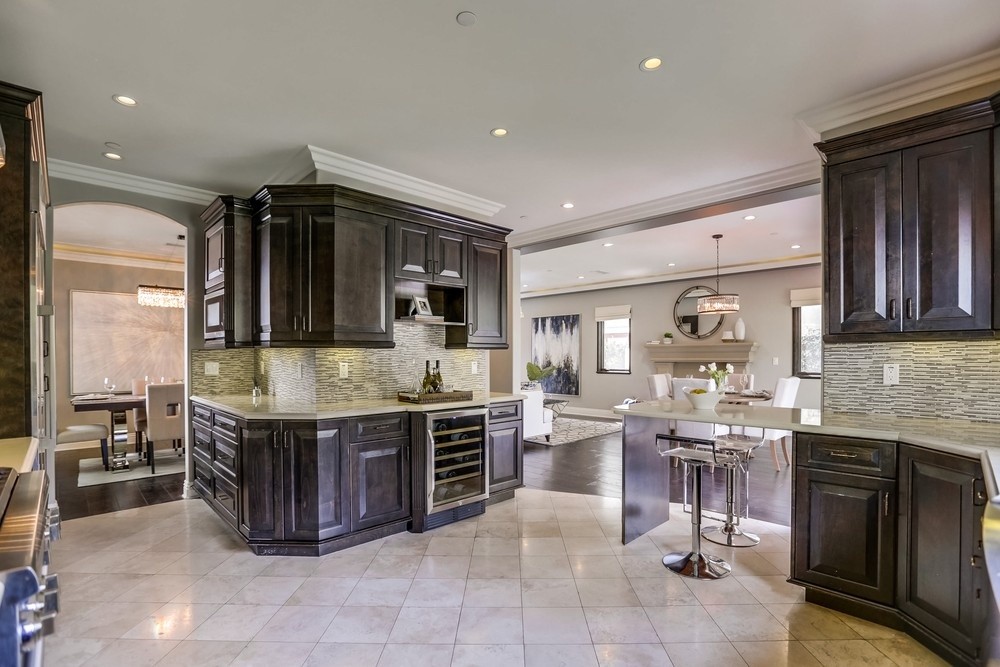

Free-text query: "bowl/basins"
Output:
<box><xmin>682</xmin><ymin>386</ymin><xmax>725</xmax><ymax>410</ymax></box>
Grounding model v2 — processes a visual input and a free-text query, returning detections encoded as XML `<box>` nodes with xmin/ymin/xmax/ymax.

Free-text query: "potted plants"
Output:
<box><xmin>662</xmin><ymin>332</ymin><xmax>674</xmax><ymax>344</ymax></box>
<box><xmin>522</xmin><ymin>360</ymin><xmax>557</xmax><ymax>390</ymax></box>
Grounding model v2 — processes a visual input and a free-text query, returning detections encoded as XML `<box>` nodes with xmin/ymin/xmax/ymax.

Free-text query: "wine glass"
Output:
<box><xmin>104</xmin><ymin>377</ymin><xmax>116</xmax><ymax>401</ymax></box>
<box><xmin>740</xmin><ymin>375</ymin><xmax>748</xmax><ymax>392</ymax></box>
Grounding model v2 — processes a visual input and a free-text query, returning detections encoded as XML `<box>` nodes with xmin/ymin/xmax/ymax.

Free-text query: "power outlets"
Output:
<box><xmin>339</xmin><ymin>362</ymin><xmax>349</xmax><ymax>380</ymax></box>
<box><xmin>881</xmin><ymin>363</ymin><xmax>901</xmax><ymax>388</ymax></box>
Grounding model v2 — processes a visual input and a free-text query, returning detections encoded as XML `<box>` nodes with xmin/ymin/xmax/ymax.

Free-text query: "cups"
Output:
<box><xmin>723</xmin><ymin>331</ymin><xmax>732</xmax><ymax>339</ymax></box>
<box><xmin>443</xmin><ymin>384</ymin><xmax>454</xmax><ymax>392</ymax></box>
<box><xmin>144</xmin><ymin>375</ymin><xmax>155</xmax><ymax>384</ymax></box>
<box><xmin>729</xmin><ymin>386</ymin><xmax>734</xmax><ymax>391</ymax></box>
<box><xmin>160</xmin><ymin>376</ymin><xmax>169</xmax><ymax>383</ymax></box>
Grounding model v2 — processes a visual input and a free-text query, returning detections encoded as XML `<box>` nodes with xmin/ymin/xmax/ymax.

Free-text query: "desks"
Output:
<box><xmin>67</xmin><ymin>392</ymin><xmax>147</xmax><ymax>471</ymax></box>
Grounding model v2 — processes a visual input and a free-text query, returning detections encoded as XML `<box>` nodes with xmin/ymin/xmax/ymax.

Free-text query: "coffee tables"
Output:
<box><xmin>542</xmin><ymin>398</ymin><xmax>570</xmax><ymax>423</ymax></box>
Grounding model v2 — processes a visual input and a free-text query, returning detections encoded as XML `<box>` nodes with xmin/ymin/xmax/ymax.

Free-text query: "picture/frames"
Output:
<box><xmin>411</xmin><ymin>294</ymin><xmax>434</xmax><ymax>316</ymax></box>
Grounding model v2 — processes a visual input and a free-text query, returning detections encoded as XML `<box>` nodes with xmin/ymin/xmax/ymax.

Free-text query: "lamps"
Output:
<box><xmin>696</xmin><ymin>233</ymin><xmax>741</xmax><ymax>316</ymax></box>
<box><xmin>135</xmin><ymin>282</ymin><xmax>186</xmax><ymax>311</ymax></box>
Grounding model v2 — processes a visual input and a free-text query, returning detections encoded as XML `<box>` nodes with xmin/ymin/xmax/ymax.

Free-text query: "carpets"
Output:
<box><xmin>76</xmin><ymin>446</ymin><xmax>185</xmax><ymax>489</ymax></box>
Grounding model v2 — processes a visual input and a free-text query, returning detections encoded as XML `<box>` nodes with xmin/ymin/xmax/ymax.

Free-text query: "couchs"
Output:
<box><xmin>514</xmin><ymin>390</ymin><xmax>554</xmax><ymax>443</ymax></box>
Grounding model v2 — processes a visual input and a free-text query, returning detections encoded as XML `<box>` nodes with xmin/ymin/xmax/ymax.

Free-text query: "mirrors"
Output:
<box><xmin>672</xmin><ymin>283</ymin><xmax>725</xmax><ymax>340</ymax></box>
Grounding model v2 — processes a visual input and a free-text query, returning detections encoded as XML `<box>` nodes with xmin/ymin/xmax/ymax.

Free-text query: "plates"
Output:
<box><xmin>725</xmin><ymin>391</ymin><xmax>738</xmax><ymax>393</ymax></box>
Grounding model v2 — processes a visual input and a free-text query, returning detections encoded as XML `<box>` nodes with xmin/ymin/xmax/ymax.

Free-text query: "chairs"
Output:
<box><xmin>670</xmin><ymin>375</ymin><xmax>719</xmax><ymax>476</ymax></box>
<box><xmin>653</xmin><ymin>432</ymin><xmax>741</xmax><ymax>582</ymax></box>
<box><xmin>131</xmin><ymin>376</ymin><xmax>163</xmax><ymax>460</ymax></box>
<box><xmin>700</xmin><ymin>425</ymin><xmax>762</xmax><ymax>549</ymax></box>
<box><xmin>144</xmin><ymin>382</ymin><xmax>186</xmax><ymax>475</ymax></box>
<box><xmin>645</xmin><ymin>372</ymin><xmax>680</xmax><ymax>470</ymax></box>
<box><xmin>730</xmin><ymin>375</ymin><xmax>801</xmax><ymax>473</ymax></box>
<box><xmin>54</xmin><ymin>421</ymin><xmax>110</xmax><ymax>474</ymax></box>
<box><xmin>726</xmin><ymin>372</ymin><xmax>755</xmax><ymax>435</ymax></box>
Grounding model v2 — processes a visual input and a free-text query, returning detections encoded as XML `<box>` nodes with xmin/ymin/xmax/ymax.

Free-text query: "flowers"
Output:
<box><xmin>698</xmin><ymin>362</ymin><xmax>734</xmax><ymax>385</ymax></box>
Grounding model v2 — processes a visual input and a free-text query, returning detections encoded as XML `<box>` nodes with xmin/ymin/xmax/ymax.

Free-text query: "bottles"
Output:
<box><xmin>423</xmin><ymin>360</ymin><xmax>434</xmax><ymax>394</ymax></box>
<box><xmin>408</xmin><ymin>369</ymin><xmax>425</xmax><ymax>401</ymax></box>
<box><xmin>433</xmin><ymin>423</ymin><xmax>471</xmax><ymax>479</ymax></box>
<box><xmin>433</xmin><ymin>360</ymin><xmax>444</xmax><ymax>393</ymax></box>
<box><xmin>735</xmin><ymin>318</ymin><xmax>745</xmax><ymax>339</ymax></box>
<box><xmin>253</xmin><ymin>386</ymin><xmax>261</xmax><ymax>397</ymax></box>
<box><xmin>431</xmin><ymin>367</ymin><xmax>436</xmax><ymax>380</ymax></box>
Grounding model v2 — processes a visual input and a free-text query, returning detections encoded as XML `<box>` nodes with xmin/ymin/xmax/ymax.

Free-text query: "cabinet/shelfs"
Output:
<box><xmin>199</xmin><ymin>217</ymin><xmax>251</xmax><ymax>353</ymax></box>
<box><xmin>284</xmin><ymin>416</ymin><xmax>349</xmax><ymax>558</ymax></box>
<box><xmin>347</xmin><ymin>412</ymin><xmax>413</xmax><ymax>550</ymax></box>
<box><xmin>444</xmin><ymin>236</ymin><xmax>510</xmax><ymax>352</ymax></box>
<box><xmin>394</xmin><ymin>219</ymin><xmax>468</xmax><ymax>289</ymax></box>
<box><xmin>484</xmin><ymin>399</ymin><xmax>526</xmax><ymax>505</ymax></box>
<box><xmin>811</xmin><ymin>96</ymin><xmax>1000</xmax><ymax>346</ymax></box>
<box><xmin>252</xmin><ymin>207</ymin><xmax>299</xmax><ymax>349</ymax></box>
<box><xmin>299</xmin><ymin>203</ymin><xmax>389</xmax><ymax>343</ymax></box>
<box><xmin>213</xmin><ymin>409</ymin><xmax>238</xmax><ymax>530</ymax></box>
<box><xmin>784</xmin><ymin>433</ymin><xmax>898</xmax><ymax>627</ymax></box>
<box><xmin>894</xmin><ymin>442</ymin><xmax>988</xmax><ymax>667</ymax></box>
<box><xmin>238</xmin><ymin>418</ymin><xmax>282</xmax><ymax>557</ymax></box>
<box><xmin>191</xmin><ymin>403</ymin><xmax>213</xmax><ymax>508</ymax></box>
